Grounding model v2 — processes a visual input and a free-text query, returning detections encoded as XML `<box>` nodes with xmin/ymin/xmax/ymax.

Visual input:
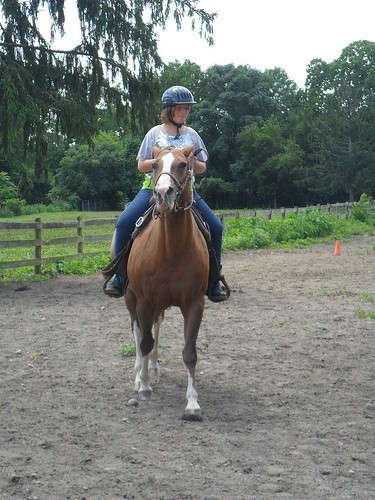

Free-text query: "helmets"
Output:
<box><xmin>161</xmin><ymin>86</ymin><xmax>197</xmax><ymax>106</ymax></box>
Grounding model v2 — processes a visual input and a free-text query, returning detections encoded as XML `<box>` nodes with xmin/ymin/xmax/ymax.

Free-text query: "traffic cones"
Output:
<box><xmin>334</xmin><ymin>239</ymin><xmax>341</xmax><ymax>256</ymax></box>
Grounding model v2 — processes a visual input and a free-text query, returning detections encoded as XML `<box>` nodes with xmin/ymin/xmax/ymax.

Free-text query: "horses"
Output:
<box><xmin>111</xmin><ymin>144</ymin><xmax>209</xmax><ymax>422</ymax></box>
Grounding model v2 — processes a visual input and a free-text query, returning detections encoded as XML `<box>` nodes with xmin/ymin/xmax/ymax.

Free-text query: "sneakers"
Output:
<box><xmin>210</xmin><ymin>279</ymin><xmax>227</xmax><ymax>299</ymax></box>
<box><xmin>105</xmin><ymin>274</ymin><xmax>122</xmax><ymax>295</ymax></box>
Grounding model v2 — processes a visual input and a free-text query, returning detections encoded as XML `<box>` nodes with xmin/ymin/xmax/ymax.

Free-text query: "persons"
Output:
<box><xmin>103</xmin><ymin>86</ymin><xmax>230</xmax><ymax>304</ymax></box>
